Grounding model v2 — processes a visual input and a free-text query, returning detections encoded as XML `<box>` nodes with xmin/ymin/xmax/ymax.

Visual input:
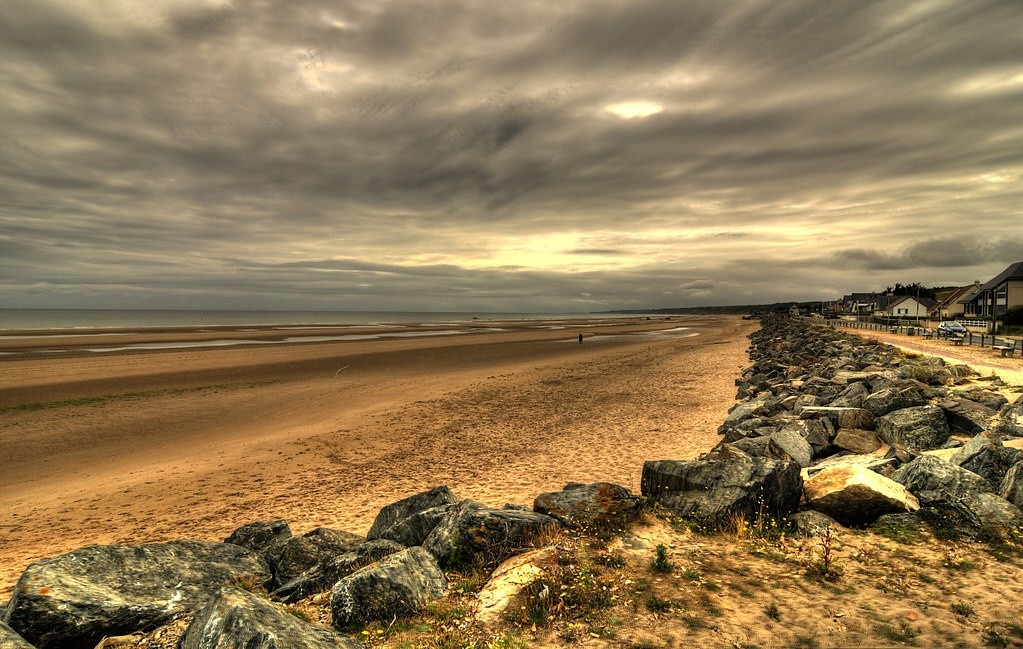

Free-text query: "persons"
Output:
<box><xmin>579</xmin><ymin>333</ymin><xmax>583</xmax><ymax>343</ymax></box>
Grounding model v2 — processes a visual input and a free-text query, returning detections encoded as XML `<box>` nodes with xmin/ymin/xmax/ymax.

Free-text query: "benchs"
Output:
<box><xmin>923</xmin><ymin>328</ymin><xmax>933</xmax><ymax>339</ymax></box>
<box><xmin>993</xmin><ymin>338</ymin><xmax>1017</xmax><ymax>357</ymax></box>
<box><xmin>948</xmin><ymin>330</ymin><xmax>967</xmax><ymax>346</ymax></box>
<box><xmin>889</xmin><ymin>326</ymin><xmax>898</xmax><ymax>334</ymax></box>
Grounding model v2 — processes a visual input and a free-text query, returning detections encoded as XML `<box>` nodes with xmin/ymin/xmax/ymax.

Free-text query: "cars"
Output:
<box><xmin>937</xmin><ymin>321</ymin><xmax>967</xmax><ymax>338</ymax></box>
